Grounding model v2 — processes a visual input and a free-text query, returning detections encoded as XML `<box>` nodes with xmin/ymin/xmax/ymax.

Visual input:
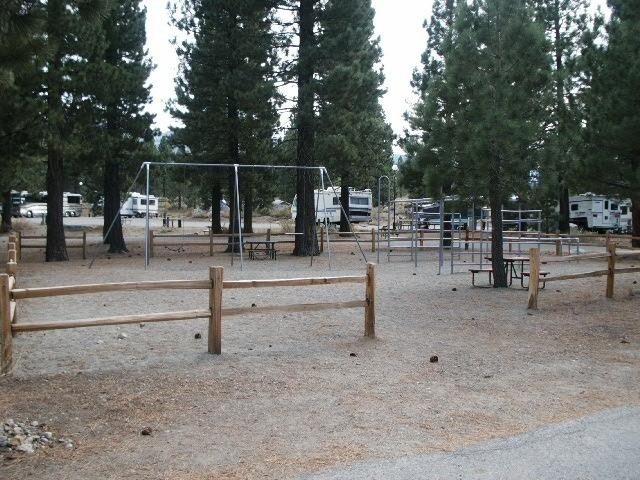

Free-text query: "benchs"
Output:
<box><xmin>248</xmin><ymin>249</ymin><xmax>280</xmax><ymax>260</ymax></box>
<box><xmin>468</xmin><ymin>269</ymin><xmax>492</xmax><ymax>288</ymax></box>
<box><xmin>520</xmin><ymin>272</ymin><xmax>550</xmax><ymax>289</ymax></box>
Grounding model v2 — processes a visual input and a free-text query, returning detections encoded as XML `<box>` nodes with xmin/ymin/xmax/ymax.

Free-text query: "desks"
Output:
<box><xmin>246</xmin><ymin>240</ymin><xmax>278</xmax><ymax>250</ymax></box>
<box><xmin>484</xmin><ymin>257</ymin><xmax>530</xmax><ymax>286</ymax></box>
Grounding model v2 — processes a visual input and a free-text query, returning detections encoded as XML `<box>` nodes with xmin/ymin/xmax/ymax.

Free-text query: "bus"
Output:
<box><xmin>21</xmin><ymin>194</ymin><xmax>82</xmax><ymax>217</ymax></box>
